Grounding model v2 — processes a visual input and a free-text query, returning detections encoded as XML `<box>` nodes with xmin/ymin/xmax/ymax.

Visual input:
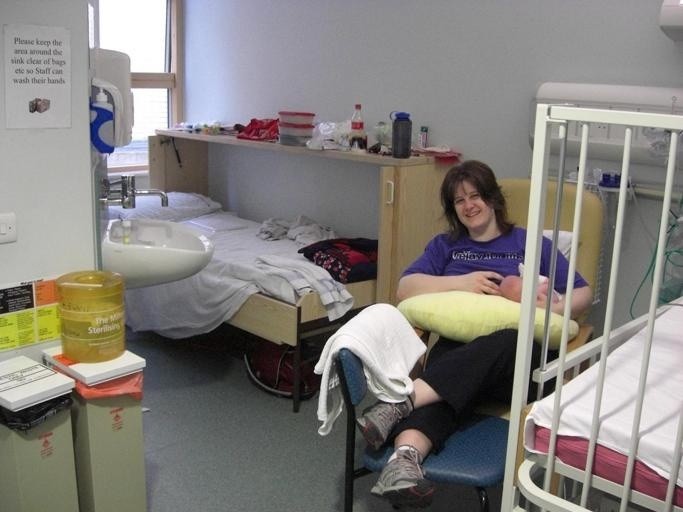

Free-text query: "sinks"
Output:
<box><xmin>101</xmin><ymin>216</ymin><xmax>213</xmax><ymax>289</ymax></box>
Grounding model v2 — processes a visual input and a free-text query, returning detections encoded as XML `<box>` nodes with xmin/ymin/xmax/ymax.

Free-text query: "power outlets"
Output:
<box><xmin>594</xmin><ymin>122</ymin><xmax>608</xmax><ymax>139</ymax></box>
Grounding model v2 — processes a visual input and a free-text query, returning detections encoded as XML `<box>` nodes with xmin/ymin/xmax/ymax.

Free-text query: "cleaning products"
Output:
<box><xmin>116</xmin><ymin>217</ymin><xmax>133</xmax><ymax>243</ymax></box>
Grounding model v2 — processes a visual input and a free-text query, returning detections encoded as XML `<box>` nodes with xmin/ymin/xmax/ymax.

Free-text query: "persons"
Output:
<box><xmin>499</xmin><ymin>275</ymin><xmax>559</xmax><ymax>303</ymax></box>
<box><xmin>356</xmin><ymin>161</ymin><xmax>593</xmax><ymax>511</ymax></box>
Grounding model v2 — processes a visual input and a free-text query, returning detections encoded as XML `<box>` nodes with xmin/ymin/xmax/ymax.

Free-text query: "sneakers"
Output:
<box><xmin>356</xmin><ymin>401</ymin><xmax>408</xmax><ymax>452</ymax></box>
<box><xmin>369</xmin><ymin>450</ymin><xmax>435</xmax><ymax>509</ymax></box>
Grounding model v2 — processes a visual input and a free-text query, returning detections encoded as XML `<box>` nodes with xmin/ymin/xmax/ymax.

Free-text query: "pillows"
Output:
<box><xmin>397</xmin><ymin>291</ymin><xmax>578</xmax><ymax>349</ymax></box>
<box><xmin>109</xmin><ymin>187</ymin><xmax>222</xmax><ymax>222</ymax></box>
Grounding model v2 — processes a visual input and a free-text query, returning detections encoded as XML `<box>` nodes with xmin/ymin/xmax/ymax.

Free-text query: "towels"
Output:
<box><xmin>310</xmin><ymin>300</ymin><xmax>427</xmax><ymax>435</ymax></box>
<box><xmin>253</xmin><ymin>253</ymin><xmax>354</xmax><ymax>323</ymax></box>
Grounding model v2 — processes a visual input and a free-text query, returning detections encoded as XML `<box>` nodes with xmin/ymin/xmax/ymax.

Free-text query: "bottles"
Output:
<box><xmin>374</xmin><ymin>121</ymin><xmax>385</xmax><ymax>145</ymax></box>
<box><xmin>90</xmin><ymin>87</ymin><xmax>116</xmax><ymax>154</ymax></box>
<box><xmin>388</xmin><ymin>110</ymin><xmax>413</xmax><ymax>160</ymax></box>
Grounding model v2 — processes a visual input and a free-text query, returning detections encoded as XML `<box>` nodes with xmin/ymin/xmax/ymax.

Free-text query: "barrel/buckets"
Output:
<box><xmin>53</xmin><ymin>269</ymin><xmax>127</xmax><ymax>363</ymax></box>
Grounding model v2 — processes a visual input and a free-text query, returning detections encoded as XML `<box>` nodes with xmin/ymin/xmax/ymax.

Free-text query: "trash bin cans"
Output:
<box><xmin>0</xmin><ymin>357</ymin><xmax>149</xmax><ymax>512</ymax></box>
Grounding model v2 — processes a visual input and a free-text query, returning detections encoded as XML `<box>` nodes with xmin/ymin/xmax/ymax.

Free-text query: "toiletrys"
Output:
<box><xmin>91</xmin><ymin>83</ymin><xmax>119</xmax><ymax>154</ymax></box>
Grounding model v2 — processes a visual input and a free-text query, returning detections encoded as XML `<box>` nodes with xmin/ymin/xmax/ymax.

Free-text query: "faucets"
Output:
<box><xmin>134</xmin><ymin>187</ymin><xmax>170</xmax><ymax>209</ymax></box>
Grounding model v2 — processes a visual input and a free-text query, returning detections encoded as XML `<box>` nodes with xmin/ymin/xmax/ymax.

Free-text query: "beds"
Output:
<box><xmin>99</xmin><ymin>194</ymin><xmax>377</xmax><ymax>412</ymax></box>
<box><xmin>497</xmin><ymin>101</ymin><xmax>682</xmax><ymax>511</ymax></box>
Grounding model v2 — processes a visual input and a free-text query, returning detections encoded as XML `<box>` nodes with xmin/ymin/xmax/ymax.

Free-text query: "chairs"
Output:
<box><xmin>331</xmin><ymin>342</ymin><xmax>507</xmax><ymax>512</ymax></box>
<box><xmin>458</xmin><ymin>173</ymin><xmax>604</xmax><ymax>501</ymax></box>
